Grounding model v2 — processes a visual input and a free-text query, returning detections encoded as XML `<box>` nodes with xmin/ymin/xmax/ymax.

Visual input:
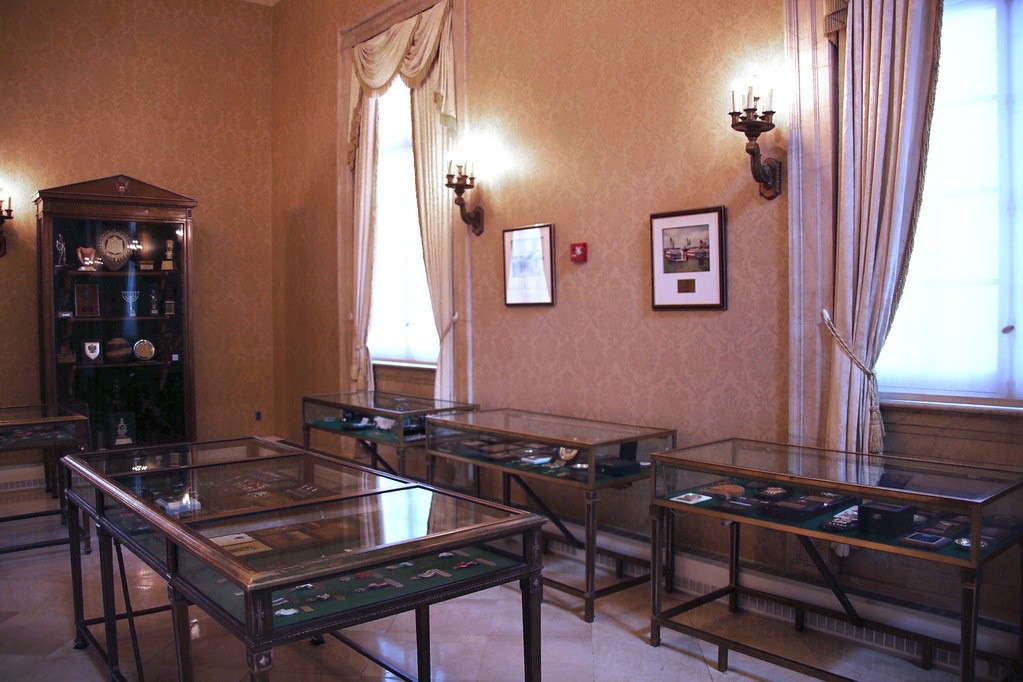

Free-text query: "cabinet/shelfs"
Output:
<box><xmin>34</xmin><ymin>174</ymin><xmax>198</xmax><ymax>501</ymax></box>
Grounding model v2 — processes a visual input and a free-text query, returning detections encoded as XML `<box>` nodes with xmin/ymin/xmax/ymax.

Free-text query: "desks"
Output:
<box><xmin>303</xmin><ymin>390</ymin><xmax>482</xmax><ymax>499</ymax></box>
<box><xmin>59</xmin><ymin>435</ymin><xmax>550</xmax><ymax>682</ymax></box>
<box><xmin>0</xmin><ymin>403</ymin><xmax>94</xmax><ymax>555</ymax></box>
<box><xmin>647</xmin><ymin>436</ymin><xmax>1023</xmax><ymax>682</ymax></box>
<box><xmin>426</xmin><ymin>406</ymin><xmax>678</xmax><ymax>624</ymax></box>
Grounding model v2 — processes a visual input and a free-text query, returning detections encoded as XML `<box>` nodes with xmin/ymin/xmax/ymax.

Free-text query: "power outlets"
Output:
<box><xmin>256</xmin><ymin>410</ymin><xmax>263</xmax><ymax>422</ymax></box>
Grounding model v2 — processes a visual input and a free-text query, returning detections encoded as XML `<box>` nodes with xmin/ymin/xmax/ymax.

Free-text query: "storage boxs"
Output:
<box><xmin>857</xmin><ymin>501</ymin><xmax>916</xmax><ymax>536</ymax></box>
<box><xmin>597</xmin><ymin>456</ymin><xmax>641</xmax><ymax>478</ymax></box>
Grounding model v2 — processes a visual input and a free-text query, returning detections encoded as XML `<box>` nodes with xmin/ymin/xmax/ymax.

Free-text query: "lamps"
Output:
<box><xmin>728</xmin><ymin>86</ymin><xmax>782</xmax><ymax>200</ymax></box>
<box><xmin>445</xmin><ymin>160</ymin><xmax>485</xmax><ymax>237</ymax></box>
<box><xmin>0</xmin><ymin>187</ymin><xmax>15</xmax><ymax>258</ymax></box>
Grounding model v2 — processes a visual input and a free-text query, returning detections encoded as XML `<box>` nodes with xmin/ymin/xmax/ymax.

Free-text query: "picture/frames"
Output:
<box><xmin>74</xmin><ymin>283</ymin><xmax>101</xmax><ymax>317</ymax></box>
<box><xmin>897</xmin><ymin>531</ymin><xmax>953</xmax><ymax>550</ymax></box>
<box><xmin>502</xmin><ymin>222</ymin><xmax>556</xmax><ymax>306</ymax></box>
<box><xmin>650</xmin><ymin>205</ymin><xmax>728</xmax><ymax>312</ymax></box>
